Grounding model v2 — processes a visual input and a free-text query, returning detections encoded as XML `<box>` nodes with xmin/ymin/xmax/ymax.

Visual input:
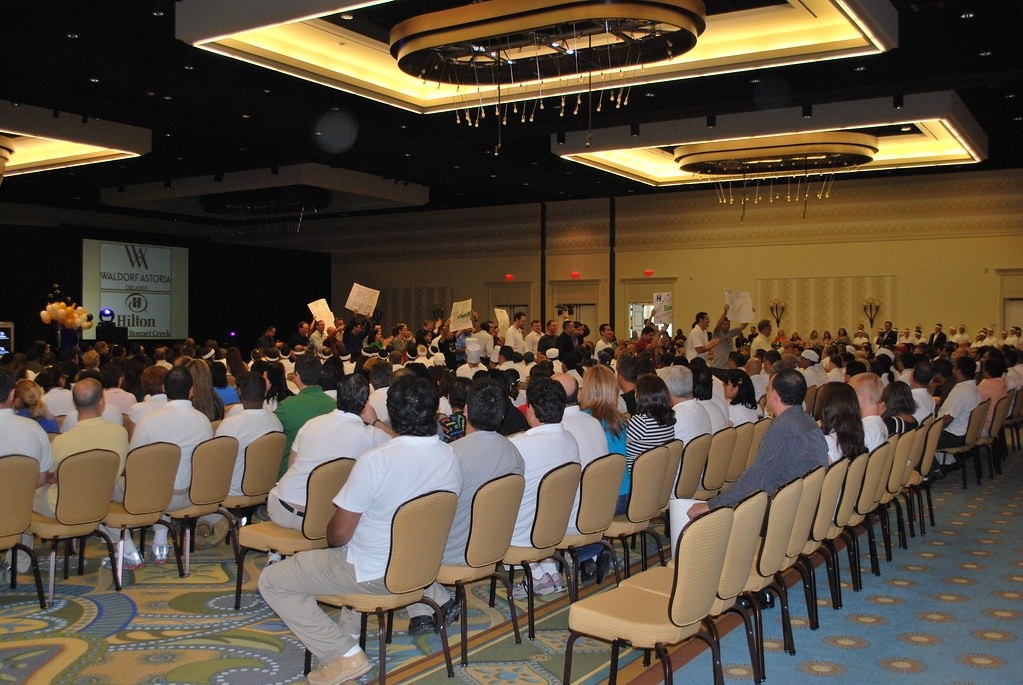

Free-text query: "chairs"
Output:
<box><xmin>0</xmin><ymin>386</ymin><xmax>1023</xmax><ymax>685</ymax></box>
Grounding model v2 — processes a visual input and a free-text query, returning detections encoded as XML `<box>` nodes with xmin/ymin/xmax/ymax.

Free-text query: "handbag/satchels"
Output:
<box><xmin>194</xmin><ymin>507</ymin><xmax>238</xmax><ymax>549</ymax></box>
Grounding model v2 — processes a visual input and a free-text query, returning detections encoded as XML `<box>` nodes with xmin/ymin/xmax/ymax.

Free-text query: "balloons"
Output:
<box><xmin>40</xmin><ymin>283</ymin><xmax>93</xmax><ymax>330</ymax></box>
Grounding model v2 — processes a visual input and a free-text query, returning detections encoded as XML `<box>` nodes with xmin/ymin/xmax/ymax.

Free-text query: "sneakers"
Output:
<box><xmin>146</xmin><ymin>542</ymin><xmax>170</xmax><ymax>565</ymax></box>
<box><xmin>553</xmin><ymin>573</ymin><xmax>567</xmax><ymax>593</ymax></box>
<box><xmin>408</xmin><ymin>615</ymin><xmax>435</xmax><ymax>636</ymax></box>
<box><xmin>306</xmin><ymin>649</ymin><xmax>373</xmax><ymax>685</ymax></box>
<box><xmin>101</xmin><ymin>545</ymin><xmax>145</xmax><ymax>570</ymax></box>
<box><xmin>432</xmin><ymin>597</ymin><xmax>460</xmax><ymax>634</ymax></box>
<box><xmin>510</xmin><ymin>572</ymin><xmax>558</xmax><ymax>600</ymax></box>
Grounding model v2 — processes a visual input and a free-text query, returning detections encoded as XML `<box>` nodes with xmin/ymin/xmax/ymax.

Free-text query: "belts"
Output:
<box><xmin>278</xmin><ymin>497</ymin><xmax>304</xmax><ymax>517</ymax></box>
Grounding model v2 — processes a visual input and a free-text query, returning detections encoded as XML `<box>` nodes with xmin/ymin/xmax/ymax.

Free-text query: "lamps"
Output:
<box><xmin>631</xmin><ymin>123</ymin><xmax>639</xmax><ymax>137</ymax></box>
<box><xmin>557</xmin><ymin>132</ymin><xmax>565</xmax><ymax>145</ymax></box>
<box><xmin>551</xmin><ymin>91</ymin><xmax>988</xmax><ymax>205</ymax></box>
<box><xmin>174</xmin><ymin>0</ymin><xmax>899</xmax><ymax>127</ymax></box>
<box><xmin>893</xmin><ymin>94</ymin><xmax>903</xmax><ymax>111</ymax></box>
<box><xmin>802</xmin><ymin>104</ymin><xmax>813</xmax><ymax>119</ymax></box>
<box><xmin>0</xmin><ymin>100</ymin><xmax>153</xmax><ymax>186</ymax></box>
<box><xmin>769</xmin><ymin>298</ymin><xmax>786</xmax><ymax>328</ymax></box>
<box><xmin>706</xmin><ymin>115</ymin><xmax>716</xmax><ymax>128</ymax></box>
<box><xmin>862</xmin><ymin>297</ymin><xmax>880</xmax><ymax>329</ymax></box>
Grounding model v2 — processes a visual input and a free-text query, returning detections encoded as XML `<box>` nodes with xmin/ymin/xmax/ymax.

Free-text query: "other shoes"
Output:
<box><xmin>265</xmin><ymin>553</ymin><xmax>281</xmax><ymax>566</ymax></box>
<box><xmin>751</xmin><ymin>591</ymin><xmax>775</xmax><ymax>610</ymax></box>
<box><xmin>595</xmin><ymin>550</ymin><xmax>611</xmax><ymax>585</ymax></box>
<box><xmin>579</xmin><ymin>559</ymin><xmax>595</xmax><ymax>584</ymax></box>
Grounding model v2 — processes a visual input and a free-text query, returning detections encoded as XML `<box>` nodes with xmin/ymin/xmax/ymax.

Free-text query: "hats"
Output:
<box><xmin>801</xmin><ymin>350</ymin><xmax>819</xmax><ymax>363</ymax></box>
<box><xmin>490</xmin><ymin>345</ymin><xmax>502</xmax><ymax>363</ymax></box>
<box><xmin>875</xmin><ymin>348</ymin><xmax>895</xmax><ymax>362</ymax></box>
<box><xmin>433</xmin><ymin>353</ymin><xmax>445</xmax><ymax>365</ymax></box>
<box><xmin>466</xmin><ymin>345</ymin><xmax>481</xmax><ymax>364</ymax></box>
<box><xmin>546</xmin><ymin>348</ymin><xmax>559</xmax><ymax>358</ymax></box>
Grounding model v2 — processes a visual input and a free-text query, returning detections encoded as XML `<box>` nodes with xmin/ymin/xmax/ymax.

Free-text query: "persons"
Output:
<box><xmin>0</xmin><ymin>304</ymin><xmax>1023</xmax><ymax>685</ymax></box>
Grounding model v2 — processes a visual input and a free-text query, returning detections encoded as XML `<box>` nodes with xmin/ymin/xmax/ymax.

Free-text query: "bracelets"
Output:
<box><xmin>372</xmin><ymin>419</ymin><xmax>380</xmax><ymax>426</ymax></box>
